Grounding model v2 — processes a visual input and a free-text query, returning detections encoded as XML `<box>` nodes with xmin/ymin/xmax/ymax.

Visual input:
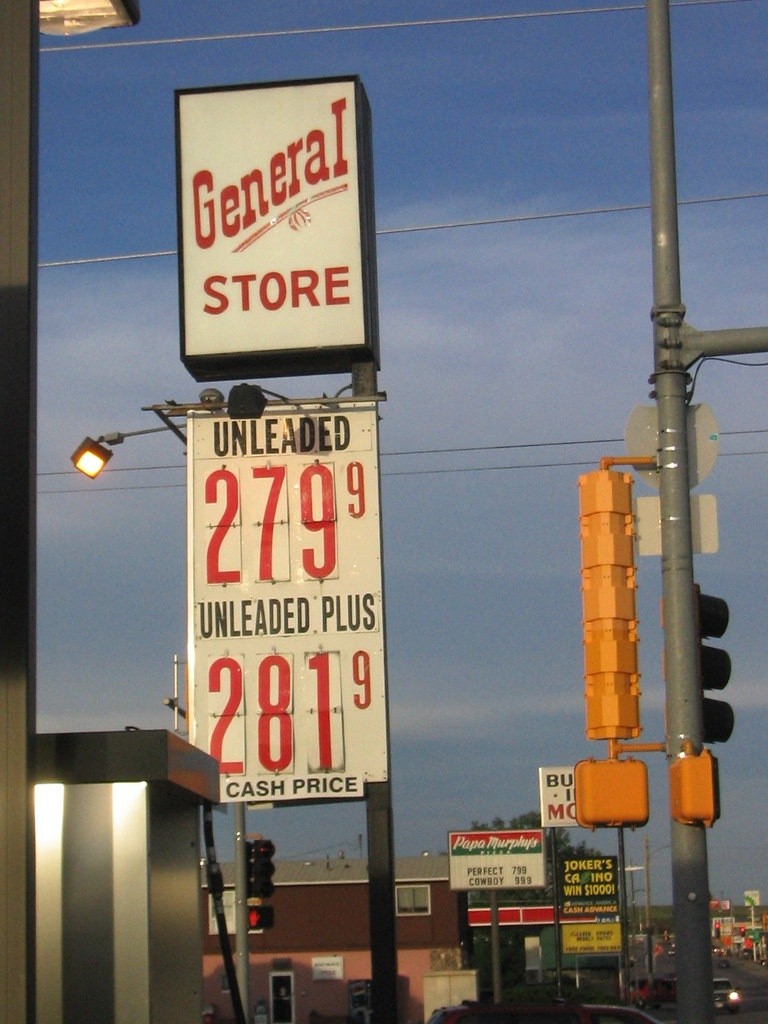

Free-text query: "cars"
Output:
<box><xmin>713</xmin><ymin>978</ymin><xmax>742</xmax><ymax>1016</ymax></box>
<box><xmin>425</xmin><ymin>998</ymin><xmax>664</xmax><ymax>1024</ymax></box>
<box><xmin>717</xmin><ymin>959</ymin><xmax>729</xmax><ymax>967</ymax></box>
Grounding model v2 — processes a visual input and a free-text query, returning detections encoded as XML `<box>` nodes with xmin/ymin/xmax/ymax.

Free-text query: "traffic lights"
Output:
<box><xmin>248</xmin><ymin>905</ymin><xmax>273</xmax><ymax>930</ymax></box>
<box><xmin>741</xmin><ymin>927</ymin><xmax>745</xmax><ymax>938</ymax></box>
<box><xmin>661</xmin><ymin>582</ymin><xmax>734</xmax><ymax>747</ymax></box>
<box><xmin>669</xmin><ymin>751</ymin><xmax>723</xmax><ymax>830</ymax></box>
<box><xmin>714</xmin><ymin>920</ymin><xmax>720</xmax><ymax>937</ymax></box>
<box><xmin>244</xmin><ymin>838</ymin><xmax>276</xmax><ymax>900</ymax></box>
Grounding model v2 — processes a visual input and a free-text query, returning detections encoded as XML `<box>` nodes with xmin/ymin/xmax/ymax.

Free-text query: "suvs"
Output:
<box><xmin>630</xmin><ymin>978</ymin><xmax>669</xmax><ymax>1009</ymax></box>
